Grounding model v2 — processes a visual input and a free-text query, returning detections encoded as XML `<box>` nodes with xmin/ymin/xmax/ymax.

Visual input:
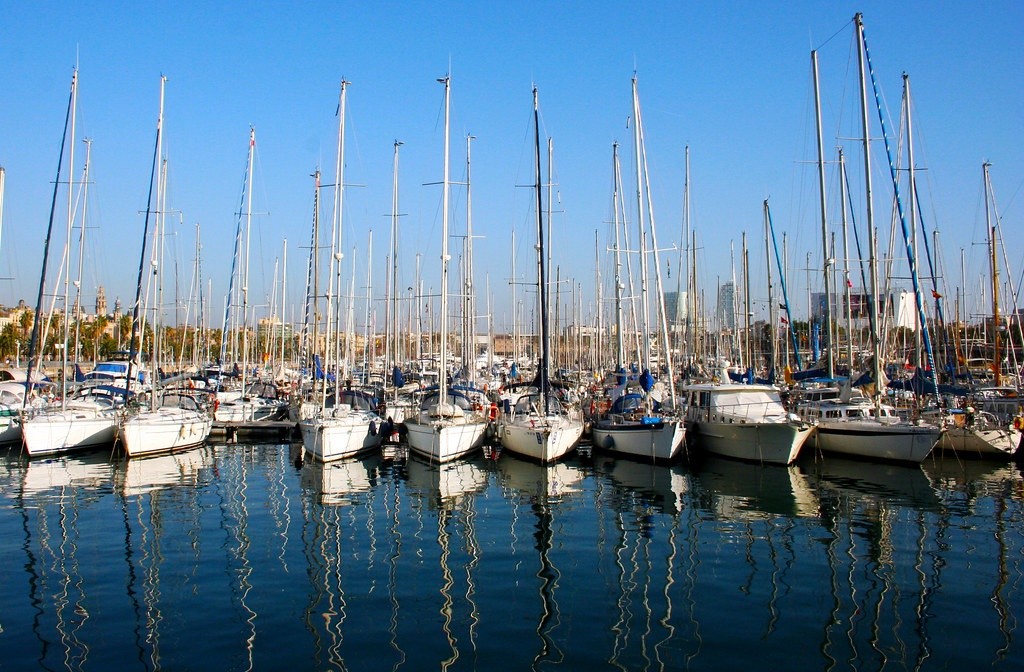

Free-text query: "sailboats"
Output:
<box><xmin>299</xmin><ymin>445</ymin><xmax>1020</xmax><ymax>672</ymax></box>
<box><xmin>1</xmin><ymin>11</ymin><xmax>1024</xmax><ymax>464</ymax></box>
<box><xmin>19</xmin><ymin>445</ymin><xmax>213</xmax><ymax>672</ymax></box>
